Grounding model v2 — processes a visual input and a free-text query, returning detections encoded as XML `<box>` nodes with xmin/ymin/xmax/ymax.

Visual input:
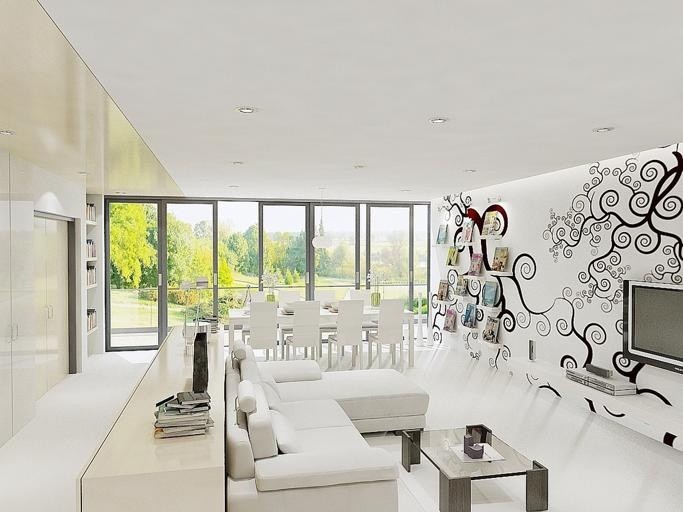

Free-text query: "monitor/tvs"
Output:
<box><xmin>622</xmin><ymin>279</ymin><xmax>683</xmax><ymax>374</ymax></box>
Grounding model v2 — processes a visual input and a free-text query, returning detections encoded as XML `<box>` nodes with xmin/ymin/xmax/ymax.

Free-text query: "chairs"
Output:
<box><xmin>242</xmin><ymin>288</ymin><xmax>406</xmax><ymax>369</ymax></box>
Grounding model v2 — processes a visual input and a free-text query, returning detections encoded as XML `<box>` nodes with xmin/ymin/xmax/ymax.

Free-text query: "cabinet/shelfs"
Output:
<box><xmin>1</xmin><ymin>148</ymin><xmax>37</xmax><ymax>447</ymax></box>
<box><xmin>86</xmin><ymin>193</ymin><xmax>106</xmax><ymax>335</ymax></box>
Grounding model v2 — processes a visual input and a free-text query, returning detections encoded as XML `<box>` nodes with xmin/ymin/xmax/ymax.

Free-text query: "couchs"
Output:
<box><xmin>226</xmin><ymin>339</ymin><xmax>431</xmax><ymax>511</ymax></box>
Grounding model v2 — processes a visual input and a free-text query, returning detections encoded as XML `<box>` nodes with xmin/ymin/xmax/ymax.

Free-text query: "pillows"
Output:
<box><xmin>260</xmin><ymin>372</ymin><xmax>302</xmax><ymax>454</ymax></box>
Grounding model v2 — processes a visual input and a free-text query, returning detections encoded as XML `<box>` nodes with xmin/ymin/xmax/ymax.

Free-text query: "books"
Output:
<box><xmin>467</xmin><ymin>252</ymin><xmax>483</xmax><ymax>277</ymax></box>
<box><xmin>443</xmin><ymin>308</ymin><xmax>457</xmax><ymax>332</ymax></box>
<box><xmin>490</xmin><ymin>246</ymin><xmax>508</xmax><ymax>272</ymax></box>
<box><xmin>434</xmin><ymin>224</ymin><xmax>448</xmax><ymax>245</ymax></box>
<box><xmin>444</xmin><ymin>245</ymin><xmax>459</xmax><ymax>267</ymax></box>
<box><xmin>481</xmin><ymin>280</ymin><xmax>498</xmax><ymax>307</ymax></box>
<box><xmin>453</xmin><ymin>275</ymin><xmax>468</xmax><ymax>297</ymax></box>
<box><xmin>436</xmin><ymin>280</ymin><xmax>449</xmax><ymax>302</ymax></box>
<box><xmin>191</xmin><ymin>314</ymin><xmax>219</xmax><ymax>333</ymax></box>
<box><xmin>459</xmin><ymin>221</ymin><xmax>474</xmax><ymax>243</ymax></box>
<box><xmin>152</xmin><ymin>390</ymin><xmax>212</xmax><ymax>439</ymax></box>
<box><xmin>481</xmin><ymin>315</ymin><xmax>500</xmax><ymax>343</ymax></box>
<box><xmin>463</xmin><ymin>303</ymin><xmax>476</xmax><ymax>328</ymax></box>
<box><xmin>480</xmin><ymin>209</ymin><xmax>497</xmax><ymax>237</ymax></box>
<box><xmin>85</xmin><ymin>200</ymin><xmax>97</xmax><ymax>330</ymax></box>
